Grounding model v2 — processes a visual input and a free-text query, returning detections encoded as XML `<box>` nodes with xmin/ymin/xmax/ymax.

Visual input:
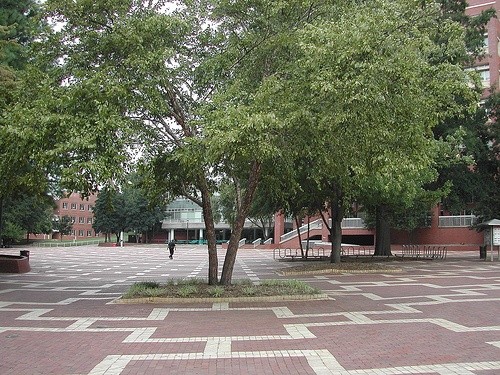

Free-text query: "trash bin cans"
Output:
<box><xmin>128</xmin><ymin>235</ymin><xmax>135</xmax><ymax>242</ymax></box>
<box><xmin>480</xmin><ymin>246</ymin><xmax>486</xmax><ymax>259</ymax></box>
<box><xmin>136</xmin><ymin>235</ymin><xmax>140</xmax><ymax>243</ymax></box>
<box><xmin>20</xmin><ymin>250</ymin><xmax>29</xmax><ymax>260</ymax></box>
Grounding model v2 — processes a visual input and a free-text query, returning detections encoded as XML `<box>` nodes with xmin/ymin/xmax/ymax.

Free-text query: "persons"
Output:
<box><xmin>167</xmin><ymin>240</ymin><xmax>177</xmax><ymax>260</ymax></box>
